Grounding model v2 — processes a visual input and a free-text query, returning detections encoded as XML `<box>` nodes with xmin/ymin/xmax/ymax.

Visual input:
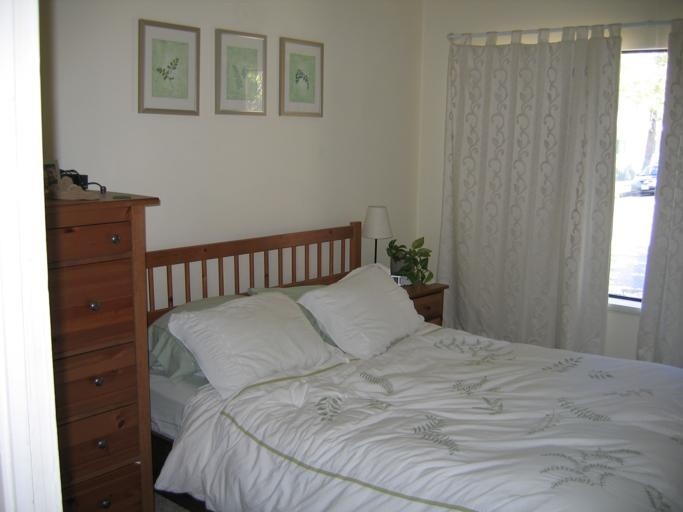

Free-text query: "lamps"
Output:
<box><xmin>361</xmin><ymin>204</ymin><xmax>394</xmax><ymax>266</ymax></box>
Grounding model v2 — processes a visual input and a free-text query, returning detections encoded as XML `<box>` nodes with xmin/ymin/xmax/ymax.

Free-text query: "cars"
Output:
<box><xmin>631</xmin><ymin>163</ymin><xmax>658</xmax><ymax>195</ymax></box>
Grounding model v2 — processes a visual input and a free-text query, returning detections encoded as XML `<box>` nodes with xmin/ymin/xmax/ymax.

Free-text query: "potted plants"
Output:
<box><xmin>387</xmin><ymin>236</ymin><xmax>435</xmax><ymax>287</ymax></box>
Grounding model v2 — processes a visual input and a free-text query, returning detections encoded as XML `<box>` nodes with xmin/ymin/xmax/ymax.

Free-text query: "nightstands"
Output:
<box><xmin>45</xmin><ymin>189</ymin><xmax>162</xmax><ymax>511</ymax></box>
<box><xmin>403</xmin><ymin>283</ymin><xmax>450</xmax><ymax>330</ymax></box>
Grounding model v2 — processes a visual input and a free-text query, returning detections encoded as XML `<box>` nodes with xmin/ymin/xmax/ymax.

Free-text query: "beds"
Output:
<box><xmin>137</xmin><ymin>218</ymin><xmax>682</xmax><ymax>511</ymax></box>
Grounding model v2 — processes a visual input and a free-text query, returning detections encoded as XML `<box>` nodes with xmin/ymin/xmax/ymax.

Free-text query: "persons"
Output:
<box><xmin>46</xmin><ymin>165</ymin><xmax>58</xmax><ymax>187</ymax></box>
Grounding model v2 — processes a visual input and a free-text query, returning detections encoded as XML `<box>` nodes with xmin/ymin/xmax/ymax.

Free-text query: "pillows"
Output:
<box><xmin>145</xmin><ymin>261</ymin><xmax>428</xmax><ymax>402</ymax></box>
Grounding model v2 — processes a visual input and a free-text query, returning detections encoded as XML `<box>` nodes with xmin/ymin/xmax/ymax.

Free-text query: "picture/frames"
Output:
<box><xmin>136</xmin><ymin>18</ymin><xmax>326</xmax><ymax>119</ymax></box>
